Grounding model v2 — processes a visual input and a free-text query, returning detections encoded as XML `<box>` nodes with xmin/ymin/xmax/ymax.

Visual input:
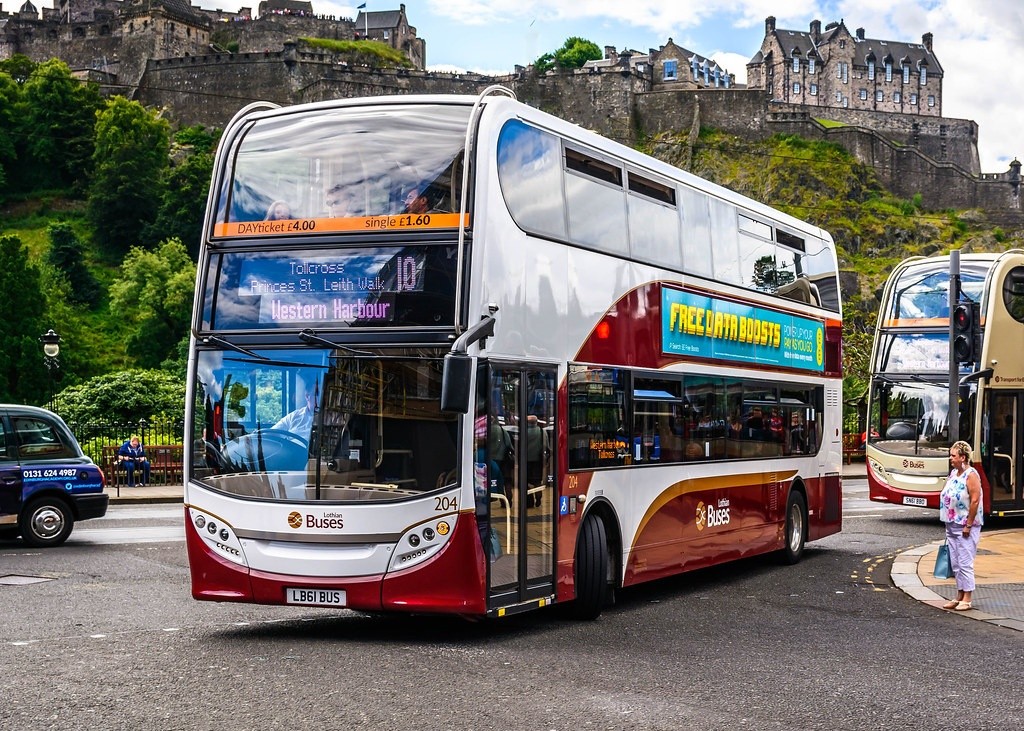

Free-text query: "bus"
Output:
<box><xmin>184</xmin><ymin>86</ymin><xmax>843</xmax><ymax>622</ymax></box>
<box><xmin>866</xmin><ymin>249</ymin><xmax>1024</xmax><ymax>518</ymax></box>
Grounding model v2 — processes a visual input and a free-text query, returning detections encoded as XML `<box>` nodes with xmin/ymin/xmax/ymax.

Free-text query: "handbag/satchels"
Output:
<box><xmin>932</xmin><ymin>537</ymin><xmax>955</xmax><ymax>580</ymax></box>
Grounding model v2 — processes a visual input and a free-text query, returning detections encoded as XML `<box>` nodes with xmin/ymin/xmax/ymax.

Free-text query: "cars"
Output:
<box><xmin>0</xmin><ymin>404</ymin><xmax>109</xmax><ymax>548</ymax></box>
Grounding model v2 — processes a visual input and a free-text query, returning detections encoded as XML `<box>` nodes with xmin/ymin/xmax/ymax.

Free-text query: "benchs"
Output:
<box><xmin>102</xmin><ymin>444</ymin><xmax>183</xmax><ymax>485</ymax></box>
<box><xmin>842</xmin><ymin>433</ymin><xmax>866</xmax><ymax>465</ymax></box>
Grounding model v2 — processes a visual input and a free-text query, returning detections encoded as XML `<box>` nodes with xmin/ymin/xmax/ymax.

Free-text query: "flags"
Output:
<box><xmin>357</xmin><ymin>3</ymin><xmax>366</xmax><ymax>9</ymax></box>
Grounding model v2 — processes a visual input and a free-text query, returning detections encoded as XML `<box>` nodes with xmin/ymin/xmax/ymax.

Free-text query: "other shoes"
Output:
<box><xmin>943</xmin><ymin>599</ymin><xmax>972</xmax><ymax>611</ymax></box>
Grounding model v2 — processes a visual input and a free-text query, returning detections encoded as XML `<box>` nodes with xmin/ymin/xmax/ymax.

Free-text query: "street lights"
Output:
<box><xmin>39</xmin><ymin>328</ymin><xmax>60</xmax><ymax>411</ymax></box>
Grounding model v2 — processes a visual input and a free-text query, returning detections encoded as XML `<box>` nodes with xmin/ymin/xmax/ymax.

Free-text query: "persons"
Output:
<box><xmin>861</xmin><ymin>425</ymin><xmax>879</xmax><ymax>449</ymax></box>
<box><xmin>195</xmin><ymin>428</ymin><xmax>206</xmax><ymax>466</ymax></box>
<box><xmin>263</xmin><ymin>382</ymin><xmax>350</xmax><ymax>459</ymax></box>
<box><xmin>491</xmin><ymin>411</ymin><xmax>549</xmax><ymax>509</ymax></box>
<box><xmin>326</xmin><ymin>184</ymin><xmax>356</xmax><ymax>218</ymax></box>
<box><xmin>119</xmin><ymin>437</ymin><xmax>151</xmax><ymax>488</ymax></box>
<box><xmin>234</xmin><ymin>7</ymin><xmax>352</xmax><ymax>22</ymax></box>
<box><xmin>405</xmin><ymin>185</ymin><xmax>442</xmax><ymax>214</ymax></box>
<box><xmin>999</xmin><ymin>415</ymin><xmax>1013</xmax><ymax>494</ymax></box>
<box><xmin>919</xmin><ymin>396</ymin><xmax>945</xmax><ymax>437</ymax></box>
<box><xmin>263</xmin><ymin>201</ymin><xmax>298</xmax><ymax>221</ymax></box>
<box><xmin>473</xmin><ymin>438</ymin><xmax>504</xmax><ymax>564</ymax></box>
<box><xmin>940</xmin><ymin>441</ymin><xmax>984</xmax><ymax>611</ymax></box>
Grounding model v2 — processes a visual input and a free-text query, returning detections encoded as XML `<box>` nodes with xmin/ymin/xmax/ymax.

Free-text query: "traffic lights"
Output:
<box><xmin>953</xmin><ymin>302</ymin><xmax>980</xmax><ymax>362</ymax></box>
